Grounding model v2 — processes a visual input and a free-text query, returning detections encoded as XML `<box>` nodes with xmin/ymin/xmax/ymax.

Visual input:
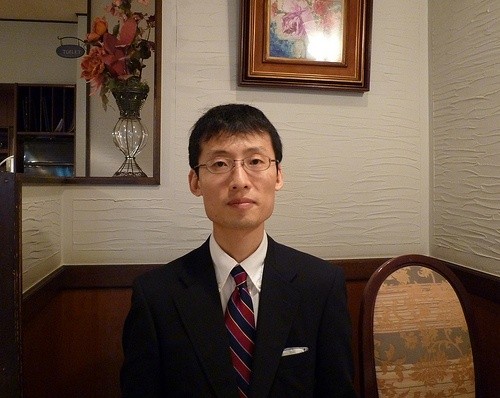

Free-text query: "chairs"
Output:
<box><xmin>360</xmin><ymin>254</ymin><xmax>481</xmax><ymax>398</ymax></box>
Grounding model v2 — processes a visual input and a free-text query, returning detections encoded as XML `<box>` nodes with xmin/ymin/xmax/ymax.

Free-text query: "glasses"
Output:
<box><xmin>194</xmin><ymin>156</ymin><xmax>279</xmax><ymax>174</ymax></box>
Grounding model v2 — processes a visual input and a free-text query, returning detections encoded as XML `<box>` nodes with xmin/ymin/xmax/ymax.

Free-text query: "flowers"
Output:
<box><xmin>80</xmin><ymin>0</ymin><xmax>156</xmax><ymax>117</ymax></box>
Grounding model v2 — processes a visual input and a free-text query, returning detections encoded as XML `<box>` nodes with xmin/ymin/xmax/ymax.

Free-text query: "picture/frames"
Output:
<box><xmin>237</xmin><ymin>0</ymin><xmax>373</xmax><ymax>91</ymax></box>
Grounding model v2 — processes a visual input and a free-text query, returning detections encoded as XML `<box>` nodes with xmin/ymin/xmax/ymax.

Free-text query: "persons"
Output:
<box><xmin>120</xmin><ymin>105</ymin><xmax>358</xmax><ymax>398</ymax></box>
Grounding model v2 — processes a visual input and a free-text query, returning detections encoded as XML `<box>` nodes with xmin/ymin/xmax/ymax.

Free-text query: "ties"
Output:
<box><xmin>224</xmin><ymin>265</ymin><xmax>256</xmax><ymax>398</ymax></box>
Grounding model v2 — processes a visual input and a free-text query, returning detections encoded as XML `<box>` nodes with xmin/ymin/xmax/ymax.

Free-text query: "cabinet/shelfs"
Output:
<box><xmin>0</xmin><ymin>82</ymin><xmax>77</xmax><ymax>176</ymax></box>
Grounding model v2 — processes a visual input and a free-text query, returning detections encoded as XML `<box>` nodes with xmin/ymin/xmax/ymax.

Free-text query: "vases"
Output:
<box><xmin>111</xmin><ymin>84</ymin><xmax>150</xmax><ymax>177</ymax></box>
